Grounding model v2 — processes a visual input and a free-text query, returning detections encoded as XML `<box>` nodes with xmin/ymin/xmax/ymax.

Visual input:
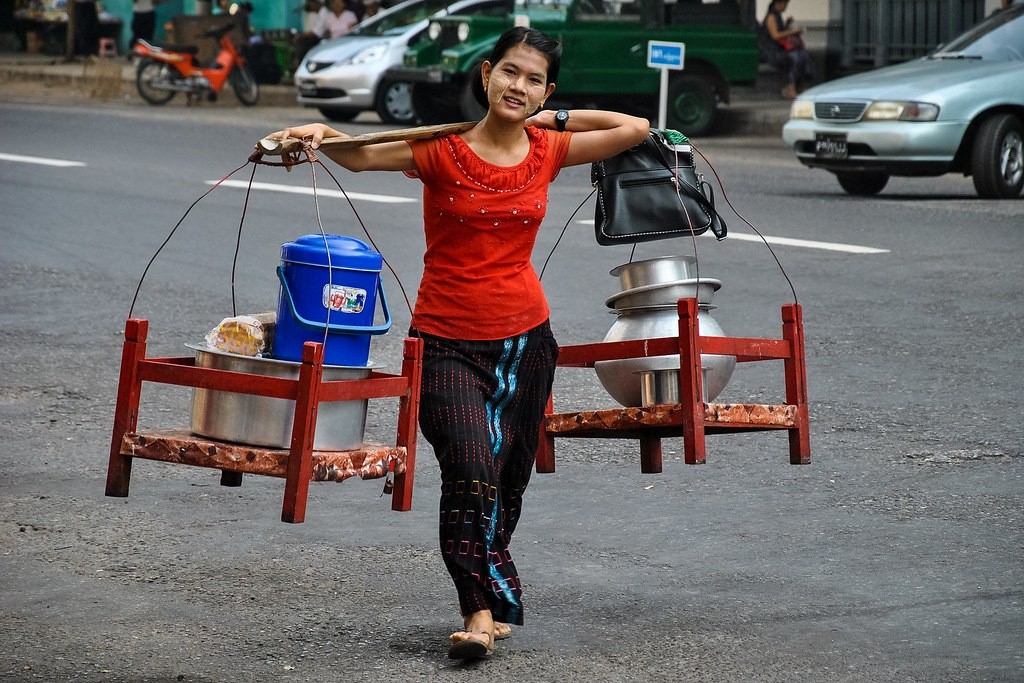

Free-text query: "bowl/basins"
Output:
<box><xmin>608</xmin><ymin>254</ymin><xmax>697</xmax><ymax>293</ymax></box>
<box><xmin>604</xmin><ymin>272</ymin><xmax>723</xmax><ymax>309</ymax></box>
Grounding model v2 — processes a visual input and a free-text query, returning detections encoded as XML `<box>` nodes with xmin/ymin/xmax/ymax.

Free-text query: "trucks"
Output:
<box><xmin>383</xmin><ymin>0</ymin><xmax>761</xmax><ymax>138</ymax></box>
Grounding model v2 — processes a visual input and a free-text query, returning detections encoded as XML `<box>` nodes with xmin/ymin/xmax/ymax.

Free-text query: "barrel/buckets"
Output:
<box><xmin>184</xmin><ymin>344</ymin><xmax>385</xmax><ymax>451</ymax></box>
<box><xmin>272</xmin><ymin>234</ymin><xmax>392</xmax><ymax>366</ymax></box>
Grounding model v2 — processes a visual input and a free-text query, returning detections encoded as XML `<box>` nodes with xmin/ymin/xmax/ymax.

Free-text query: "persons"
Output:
<box><xmin>254</xmin><ymin>26</ymin><xmax>650</xmax><ymax>660</ymax></box>
<box><xmin>760</xmin><ymin>0</ymin><xmax>802</xmax><ymax>100</ymax></box>
<box><xmin>292</xmin><ymin>0</ymin><xmax>385</xmax><ymax>86</ymax></box>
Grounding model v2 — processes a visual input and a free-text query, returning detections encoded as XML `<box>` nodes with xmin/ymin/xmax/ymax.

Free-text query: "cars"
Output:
<box><xmin>782</xmin><ymin>0</ymin><xmax>1024</xmax><ymax>201</ymax></box>
<box><xmin>294</xmin><ymin>0</ymin><xmax>514</xmax><ymax>125</ymax></box>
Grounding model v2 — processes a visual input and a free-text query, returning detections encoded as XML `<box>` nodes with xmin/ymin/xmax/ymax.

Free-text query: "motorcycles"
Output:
<box><xmin>132</xmin><ymin>0</ymin><xmax>262</xmax><ymax>111</ymax></box>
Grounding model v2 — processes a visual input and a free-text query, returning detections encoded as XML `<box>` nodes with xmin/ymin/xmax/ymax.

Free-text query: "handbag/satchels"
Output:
<box><xmin>590</xmin><ymin>124</ymin><xmax>712</xmax><ymax>246</ymax></box>
<box><xmin>777</xmin><ymin>23</ymin><xmax>804</xmax><ymax>52</ymax></box>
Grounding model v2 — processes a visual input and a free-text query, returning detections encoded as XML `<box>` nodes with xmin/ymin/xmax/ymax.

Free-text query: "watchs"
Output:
<box><xmin>555</xmin><ymin>108</ymin><xmax>571</xmax><ymax>131</ymax></box>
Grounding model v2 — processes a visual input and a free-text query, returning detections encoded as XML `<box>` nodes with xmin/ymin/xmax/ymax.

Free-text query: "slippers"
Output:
<box><xmin>494</xmin><ymin>622</ymin><xmax>511</xmax><ymax>640</ymax></box>
<box><xmin>447</xmin><ymin>628</ymin><xmax>491</xmax><ymax>661</ymax></box>
